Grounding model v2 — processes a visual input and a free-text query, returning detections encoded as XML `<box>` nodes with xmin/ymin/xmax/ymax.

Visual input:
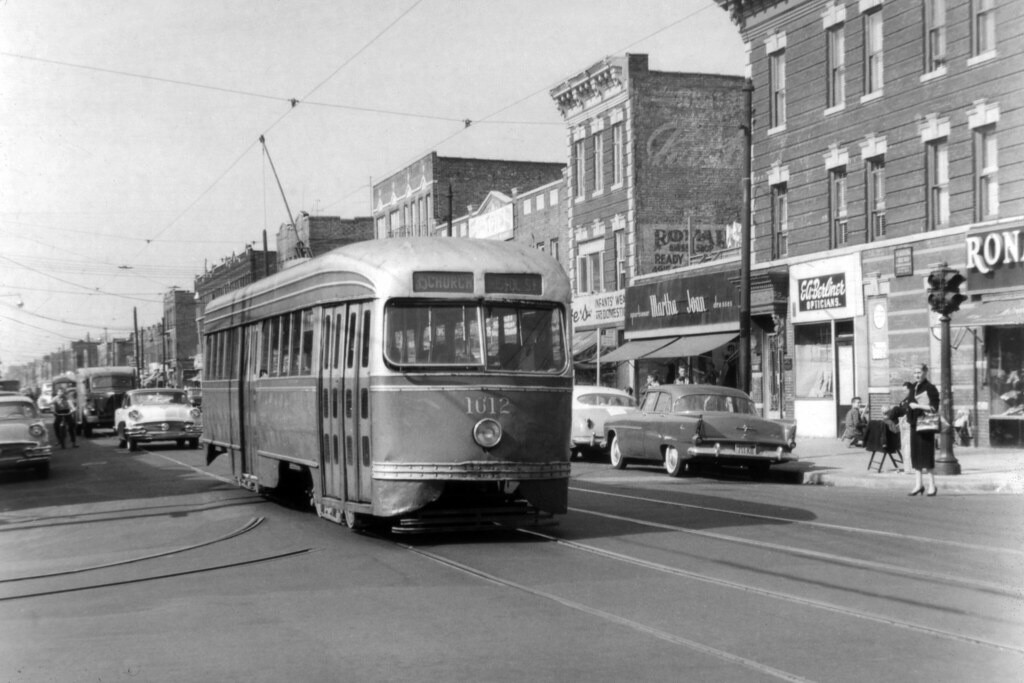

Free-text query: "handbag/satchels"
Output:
<box><xmin>916</xmin><ymin>405</ymin><xmax>941</xmax><ymax>433</ymax></box>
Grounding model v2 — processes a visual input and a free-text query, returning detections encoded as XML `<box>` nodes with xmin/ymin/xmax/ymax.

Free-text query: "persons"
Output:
<box><xmin>645</xmin><ymin>374</ymin><xmax>660</xmax><ymax>389</ymax></box>
<box><xmin>673</xmin><ymin>364</ymin><xmax>699</xmax><ymax>385</ymax></box>
<box><xmin>843</xmin><ymin>397</ymin><xmax>867</xmax><ymax>448</ymax></box>
<box><xmin>907</xmin><ymin>363</ymin><xmax>941</xmax><ymax>497</ymax></box>
<box><xmin>703</xmin><ymin>362</ymin><xmax>720</xmax><ymax>385</ymax></box>
<box><xmin>622</xmin><ymin>386</ymin><xmax>633</xmax><ymax>407</ymax></box>
<box><xmin>47</xmin><ymin>387</ymin><xmax>80</xmax><ymax>448</ymax></box>
<box><xmin>898</xmin><ymin>381</ymin><xmax>913</xmax><ymax>413</ymax></box>
<box><xmin>883</xmin><ymin>406</ymin><xmax>903</xmax><ymax>454</ymax></box>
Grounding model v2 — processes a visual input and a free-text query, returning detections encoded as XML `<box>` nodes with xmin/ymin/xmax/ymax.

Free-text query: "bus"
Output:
<box><xmin>201</xmin><ymin>235</ymin><xmax>574</xmax><ymax>531</ymax></box>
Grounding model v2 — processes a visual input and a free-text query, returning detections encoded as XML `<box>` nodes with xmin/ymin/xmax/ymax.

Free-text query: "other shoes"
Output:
<box><xmin>55</xmin><ymin>440</ymin><xmax>61</xmax><ymax>446</ymax></box>
<box><xmin>72</xmin><ymin>444</ymin><xmax>80</xmax><ymax>448</ymax></box>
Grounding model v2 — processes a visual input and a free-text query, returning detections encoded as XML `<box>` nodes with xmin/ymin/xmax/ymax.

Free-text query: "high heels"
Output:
<box><xmin>927</xmin><ymin>488</ymin><xmax>937</xmax><ymax>496</ymax></box>
<box><xmin>908</xmin><ymin>486</ymin><xmax>924</xmax><ymax>496</ymax></box>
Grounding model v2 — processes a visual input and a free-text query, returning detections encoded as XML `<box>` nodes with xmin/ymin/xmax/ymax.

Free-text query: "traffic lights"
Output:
<box><xmin>926</xmin><ymin>269</ymin><xmax>945</xmax><ymax>313</ymax></box>
<box><xmin>944</xmin><ymin>269</ymin><xmax>968</xmax><ymax>312</ymax></box>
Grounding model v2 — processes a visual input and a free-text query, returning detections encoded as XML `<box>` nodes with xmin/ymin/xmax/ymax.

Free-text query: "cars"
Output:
<box><xmin>0</xmin><ymin>390</ymin><xmax>52</xmax><ymax>481</ymax></box>
<box><xmin>113</xmin><ymin>389</ymin><xmax>204</xmax><ymax>450</ymax></box>
<box><xmin>570</xmin><ymin>387</ymin><xmax>637</xmax><ymax>459</ymax></box>
<box><xmin>603</xmin><ymin>384</ymin><xmax>798</xmax><ymax>477</ymax></box>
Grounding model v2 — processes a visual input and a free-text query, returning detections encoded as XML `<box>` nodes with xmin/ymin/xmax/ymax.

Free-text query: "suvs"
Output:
<box><xmin>70</xmin><ymin>368</ymin><xmax>141</xmax><ymax>437</ymax></box>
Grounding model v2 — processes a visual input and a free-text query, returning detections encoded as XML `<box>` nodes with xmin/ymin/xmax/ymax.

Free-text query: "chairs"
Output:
<box><xmin>416</xmin><ymin>349</ymin><xmax>429</xmax><ymax>363</ymax></box>
<box><xmin>865</xmin><ymin>419</ymin><xmax>903</xmax><ymax>473</ymax></box>
<box><xmin>953</xmin><ymin>407</ymin><xmax>971</xmax><ymax>446</ymax></box>
<box><xmin>497</xmin><ymin>342</ymin><xmax>522</xmax><ymax>370</ymax></box>
<box><xmin>386</xmin><ymin>347</ymin><xmax>401</xmax><ymax>364</ymax></box>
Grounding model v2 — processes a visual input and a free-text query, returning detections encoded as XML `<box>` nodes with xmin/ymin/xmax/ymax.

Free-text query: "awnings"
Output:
<box><xmin>588</xmin><ymin>337</ymin><xmax>678</xmax><ymax>368</ymax></box>
<box><xmin>644</xmin><ymin>332</ymin><xmax>741</xmax><ymax>362</ymax></box>
